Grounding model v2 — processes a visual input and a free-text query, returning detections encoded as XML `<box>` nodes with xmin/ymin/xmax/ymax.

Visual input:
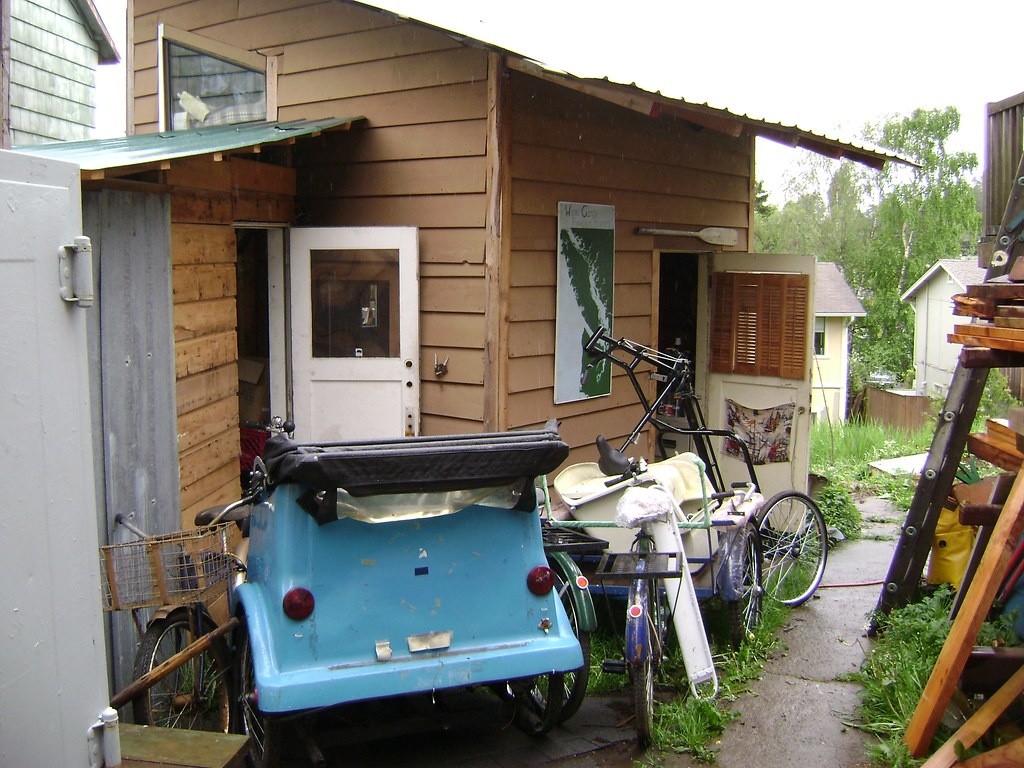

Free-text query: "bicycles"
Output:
<box><xmin>98</xmin><ymin>321</ymin><xmax>832</xmax><ymax>768</ymax></box>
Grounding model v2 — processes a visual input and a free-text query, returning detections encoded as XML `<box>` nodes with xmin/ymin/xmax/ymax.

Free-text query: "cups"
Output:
<box><xmin>658</xmin><ymin>404</ymin><xmax>674</xmax><ymax>416</ymax></box>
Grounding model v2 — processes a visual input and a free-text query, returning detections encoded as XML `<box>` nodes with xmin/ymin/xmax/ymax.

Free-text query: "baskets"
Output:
<box><xmin>99</xmin><ymin>521</ymin><xmax>237</xmax><ymax>612</ymax></box>
